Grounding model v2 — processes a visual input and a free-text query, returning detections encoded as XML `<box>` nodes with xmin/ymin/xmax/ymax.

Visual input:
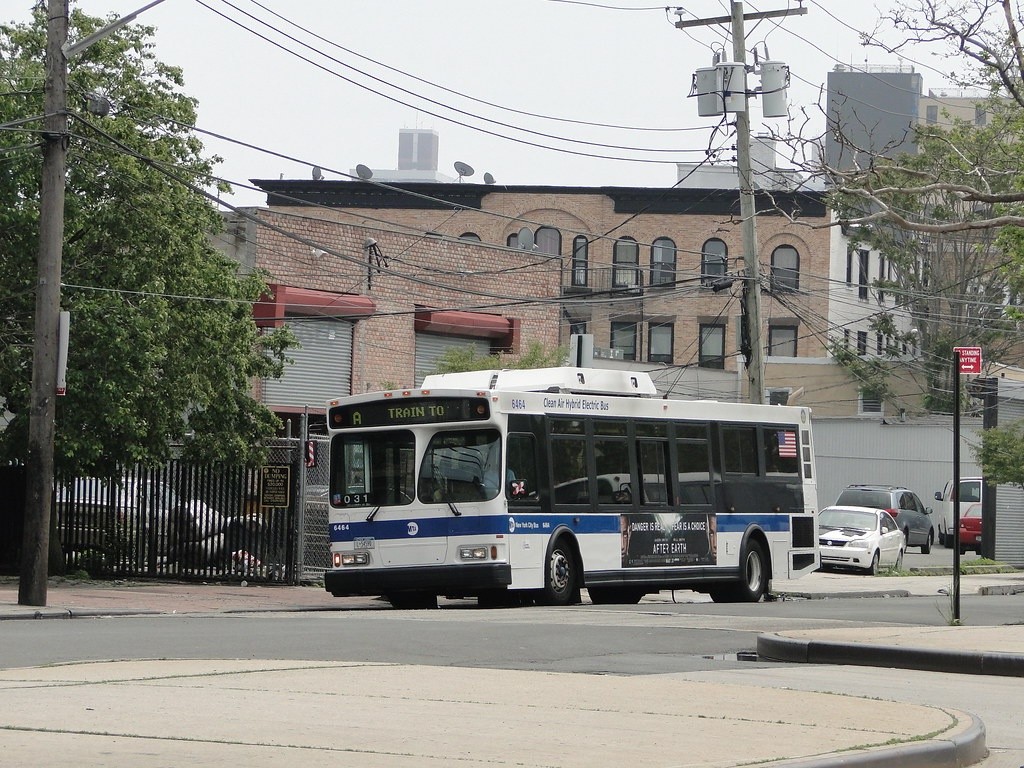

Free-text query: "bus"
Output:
<box><xmin>323</xmin><ymin>367</ymin><xmax>821</xmax><ymax>610</ymax></box>
<box><xmin>554</xmin><ymin>470</ymin><xmax>804</xmax><ymax>505</ymax></box>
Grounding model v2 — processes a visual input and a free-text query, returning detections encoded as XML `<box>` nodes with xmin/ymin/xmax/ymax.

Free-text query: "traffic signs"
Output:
<box><xmin>955</xmin><ymin>346</ymin><xmax>982</xmax><ymax>375</ymax></box>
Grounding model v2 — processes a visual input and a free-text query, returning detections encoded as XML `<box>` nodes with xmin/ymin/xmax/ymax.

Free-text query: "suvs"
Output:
<box><xmin>834</xmin><ymin>484</ymin><xmax>935</xmax><ymax>554</ymax></box>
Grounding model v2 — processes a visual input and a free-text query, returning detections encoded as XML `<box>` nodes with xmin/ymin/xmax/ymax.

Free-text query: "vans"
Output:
<box><xmin>934</xmin><ymin>476</ymin><xmax>983</xmax><ymax>549</ymax></box>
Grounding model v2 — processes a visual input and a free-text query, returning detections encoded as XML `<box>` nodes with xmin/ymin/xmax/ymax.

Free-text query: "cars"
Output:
<box><xmin>55</xmin><ymin>476</ymin><xmax>180</xmax><ymax>556</ymax></box>
<box><xmin>957</xmin><ymin>502</ymin><xmax>982</xmax><ymax>555</ymax></box>
<box><xmin>819</xmin><ymin>505</ymin><xmax>906</xmax><ymax>577</ymax></box>
